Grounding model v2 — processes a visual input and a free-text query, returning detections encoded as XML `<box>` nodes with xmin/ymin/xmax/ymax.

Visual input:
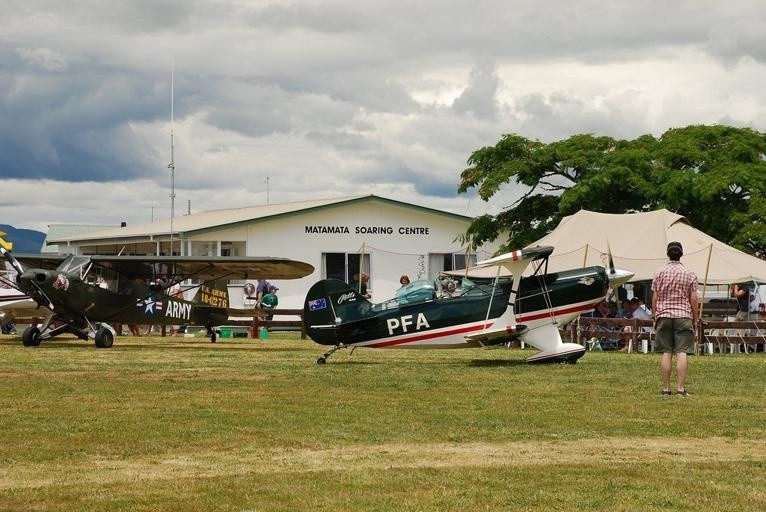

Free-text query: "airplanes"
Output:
<box><xmin>303</xmin><ymin>235</ymin><xmax>635</xmax><ymax>365</ymax></box>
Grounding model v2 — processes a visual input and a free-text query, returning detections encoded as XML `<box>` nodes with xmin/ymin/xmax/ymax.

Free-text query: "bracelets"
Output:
<box><xmin>256</xmin><ymin>299</ymin><xmax>260</xmax><ymax>303</ymax></box>
<box><xmin>652</xmin><ymin>316</ymin><xmax>656</xmax><ymax>321</ymax></box>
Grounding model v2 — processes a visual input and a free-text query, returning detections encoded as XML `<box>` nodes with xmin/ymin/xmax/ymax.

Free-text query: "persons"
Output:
<box><xmin>442</xmin><ymin>278</ymin><xmax>466</xmax><ymax>297</ymax></box>
<box><xmin>112</xmin><ymin>323</ymin><xmax>129</xmax><ymax>337</ymax></box>
<box><xmin>577</xmin><ymin>280</ymin><xmax>652</xmax><ymax>353</ymax></box>
<box><xmin>393</xmin><ymin>276</ymin><xmax>414</xmax><ymax>307</ymax></box>
<box><xmin>651</xmin><ymin>241</ymin><xmax>699</xmax><ymax>396</ymax></box>
<box><xmin>252</xmin><ymin>279</ymin><xmax>270</xmax><ymax>337</ymax></box>
<box><xmin>260</xmin><ymin>286</ymin><xmax>279</xmax><ymax>331</ymax></box>
<box><xmin>730</xmin><ymin>284</ymin><xmax>750</xmax><ymax>321</ymax></box>
<box><xmin>355</xmin><ymin>272</ymin><xmax>371</xmax><ymax>299</ymax></box>
<box><xmin>144</xmin><ymin>324</ymin><xmax>163</xmax><ymax>337</ymax></box>
<box><xmin>751</xmin><ymin>294</ymin><xmax>759</xmax><ymax>315</ymax></box>
<box><xmin>169</xmin><ymin>279</ymin><xmax>184</xmax><ymax>337</ymax></box>
<box><xmin>126</xmin><ymin>323</ymin><xmax>142</xmax><ymax>336</ymax></box>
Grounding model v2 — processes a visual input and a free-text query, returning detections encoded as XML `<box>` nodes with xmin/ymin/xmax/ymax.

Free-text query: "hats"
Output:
<box><xmin>629</xmin><ymin>297</ymin><xmax>639</xmax><ymax>304</ymax></box>
<box><xmin>270</xmin><ymin>286</ymin><xmax>279</xmax><ymax>290</ymax></box>
<box><xmin>667</xmin><ymin>242</ymin><xmax>682</xmax><ymax>252</ymax></box>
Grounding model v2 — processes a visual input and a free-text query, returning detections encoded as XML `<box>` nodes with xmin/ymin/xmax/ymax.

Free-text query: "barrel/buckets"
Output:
<box><xmin>260</xmin><ymin>328</ymin><xmax>267</xmax><ymax>339</ymax></box>
<box><xmin>186</xmin><ymin>325</ymin><xmax>205</xmax><ymax>334</ymax></box>
<box><xmin>703</xmin><ymin>342</ymin><xmax>713</xmax><ymax>353</ymax></box>
<box><xmin>221</xmin><ymin>327</ymin><xmax>230</xmax><ymax>337</ymax></box>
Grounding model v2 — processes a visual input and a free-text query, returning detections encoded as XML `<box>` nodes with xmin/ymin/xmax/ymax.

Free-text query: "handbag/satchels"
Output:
<box><xmin>264</xmin><ymin>282</ymin><xmax>271</xmax><ymax>293</ymax></box>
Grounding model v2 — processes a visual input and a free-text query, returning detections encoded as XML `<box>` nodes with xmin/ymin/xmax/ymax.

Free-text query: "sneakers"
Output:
<box><xmin>661</xmin><ymin>390</ymin><xmax>672</xmax><ymax>396</ymax></box>
<box><xmin>676</xmin><ymin>390</ymin><xmax>689</xmax><ymax>397</ymax></box>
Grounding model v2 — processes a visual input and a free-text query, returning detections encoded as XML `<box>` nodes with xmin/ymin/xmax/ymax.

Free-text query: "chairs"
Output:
<box><xmin>704</xmin><ymin>328</ymin><xmax>766</xmax><ymax>356</ymax></box>
